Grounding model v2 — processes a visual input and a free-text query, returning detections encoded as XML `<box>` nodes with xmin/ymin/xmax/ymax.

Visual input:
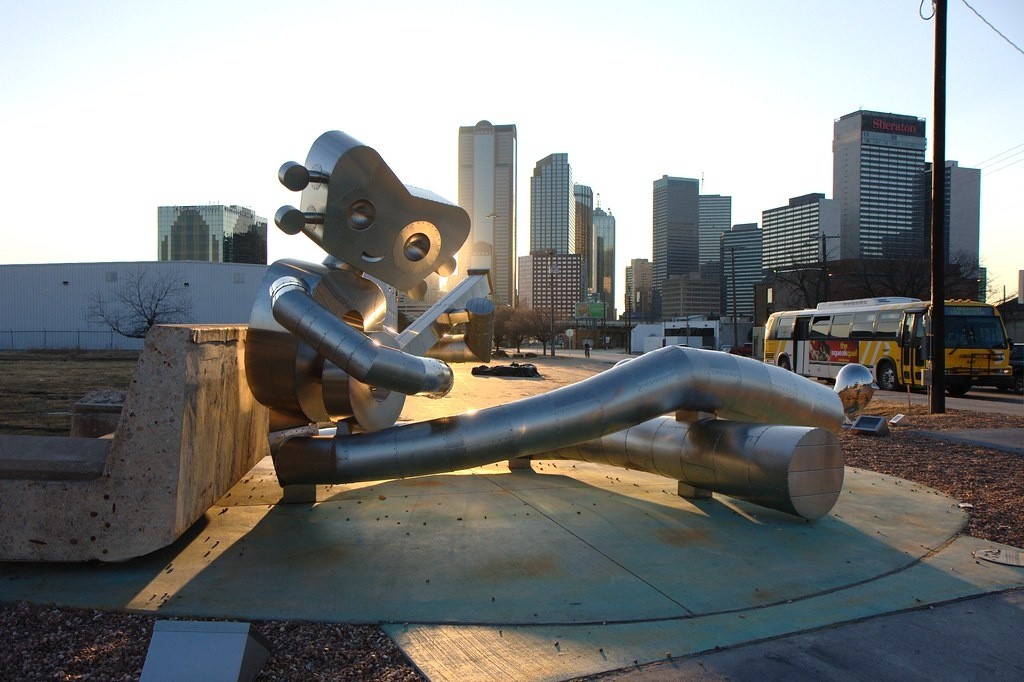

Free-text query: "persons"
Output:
<box><xmin>585</xmin><ymin>342</ymin><xmax>591</xmax><ymax>357</ymax></box>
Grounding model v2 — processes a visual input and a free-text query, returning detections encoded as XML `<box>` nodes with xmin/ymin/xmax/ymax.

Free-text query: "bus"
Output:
<box><xmin>762</xmin><ymin>296</ymin><xmax>1017</xmax><ymax>397</ymax></box>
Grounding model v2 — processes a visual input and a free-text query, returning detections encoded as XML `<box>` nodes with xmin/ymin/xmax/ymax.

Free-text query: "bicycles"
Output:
<box><xmin>585</xmin><ymin>347</ymin><xmax>590</xmax><ymax>359</ymax></box>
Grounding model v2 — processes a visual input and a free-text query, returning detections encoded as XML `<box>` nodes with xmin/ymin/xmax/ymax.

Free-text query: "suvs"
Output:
<box><xmin>996</xmin><ymin>343</ymin><xmax>1024</xmax><ymax>393</ymax></box>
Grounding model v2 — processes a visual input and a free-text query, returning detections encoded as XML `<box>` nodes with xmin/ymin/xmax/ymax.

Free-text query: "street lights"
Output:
<box><xmin>729</xmin><ymin>245</ymin><xmax>747</xmax><ymax>349</ymax></box>
